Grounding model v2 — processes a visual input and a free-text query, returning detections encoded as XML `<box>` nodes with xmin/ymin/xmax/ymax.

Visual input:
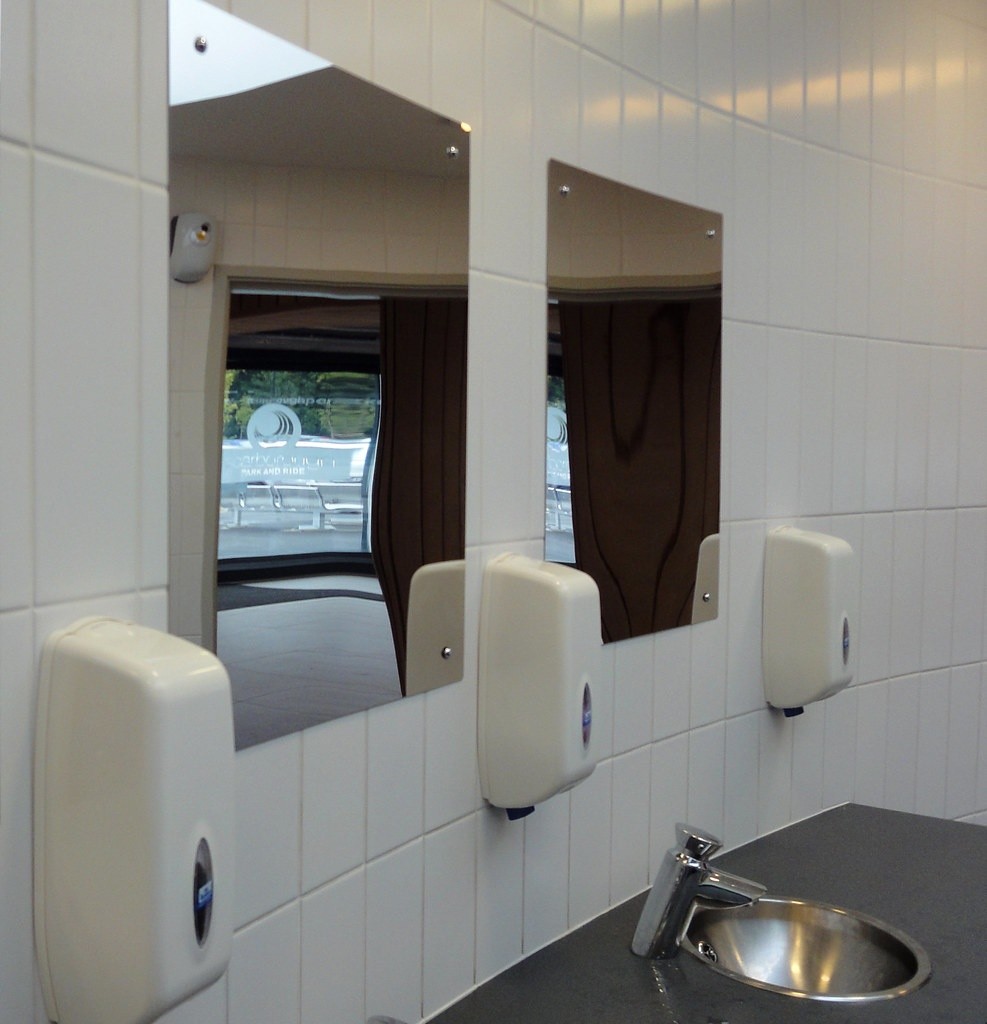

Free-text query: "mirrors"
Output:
<box><xmin>165</xmin><ymin>0</ymin><xmax>471</xmax><ymax>756</ymax></box>
<box><xmin>544</xmin><ymin>156</ymin><xmax>725</xmax><ymax>640</ymax></box>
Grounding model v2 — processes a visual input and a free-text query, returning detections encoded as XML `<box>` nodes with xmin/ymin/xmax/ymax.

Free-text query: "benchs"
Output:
<box><xmin>226</xmin><ymin>486</ymin><xmax>363</xmax><ymax>532</ymax></box>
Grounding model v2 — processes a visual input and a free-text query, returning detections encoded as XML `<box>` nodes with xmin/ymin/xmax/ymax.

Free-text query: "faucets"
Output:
<box><xmin>630</xmin><ymin>822</ymin><xmax>769</xmax><ymax>961</ymax></box>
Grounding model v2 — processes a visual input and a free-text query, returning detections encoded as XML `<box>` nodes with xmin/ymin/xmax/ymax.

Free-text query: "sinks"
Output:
<box><xmin>678</xmin><ymin>893</ymin><xmax>935</xmax><ymax>1004</ymax></box>
